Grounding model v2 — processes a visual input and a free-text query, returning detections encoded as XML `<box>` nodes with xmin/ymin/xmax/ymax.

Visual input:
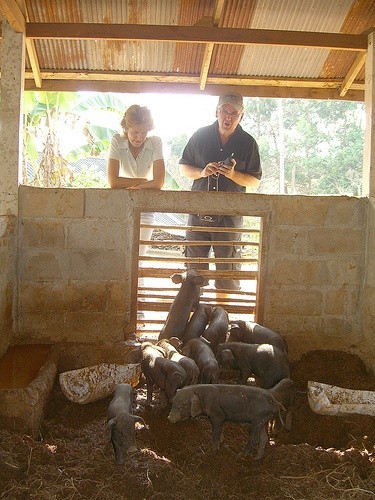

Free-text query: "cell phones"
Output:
<box><xmin>223</xmin><ymin>151</ymin><xmax>234</xmax><ymax>164</ymax></box>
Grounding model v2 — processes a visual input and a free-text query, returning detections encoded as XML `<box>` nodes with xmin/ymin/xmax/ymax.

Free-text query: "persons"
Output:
<box><xmin>107</xmin><ymin>104</ymin><xmax>166</xmax><ymax>329</ymax></box>
<box><xmin>178</xmin><ymin>91</ymin><xmax>262</xmax><ymax>305</ymax></box>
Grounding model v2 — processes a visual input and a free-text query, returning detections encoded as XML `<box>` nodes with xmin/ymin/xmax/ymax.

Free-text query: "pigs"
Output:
<box><xmin>141</xmin><ymin>268</ymin><xmax>290</xmax><ymax>410</ymax></box>
<box><xmin>108</xmin><ymin>382</ymin><xmax>146</xmax><ymax>466</ymax></box>
<box><xmin>166</xmin><ymin>384</ymin><xmax>287</xmax><ymax>463</ymax></box>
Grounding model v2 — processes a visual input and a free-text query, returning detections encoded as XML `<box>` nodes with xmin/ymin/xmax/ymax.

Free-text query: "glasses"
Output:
<box><xmin>219</xmin><ymin>109</ymin><xmax>241</xmax><ymax>118</ymax></box>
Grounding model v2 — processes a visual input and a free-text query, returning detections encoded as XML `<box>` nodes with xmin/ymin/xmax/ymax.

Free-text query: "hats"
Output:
<box><xmin>217</xmin><ymin>94</ymin><xmax>243</xmax><ymax>112</ymax></box>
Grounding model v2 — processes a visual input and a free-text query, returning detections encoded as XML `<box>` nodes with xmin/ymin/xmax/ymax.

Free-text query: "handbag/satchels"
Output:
<box><xmin>198</xmin><ymin>214</ymin><xmax>223</xmax><ymax>226</ymax></box>
